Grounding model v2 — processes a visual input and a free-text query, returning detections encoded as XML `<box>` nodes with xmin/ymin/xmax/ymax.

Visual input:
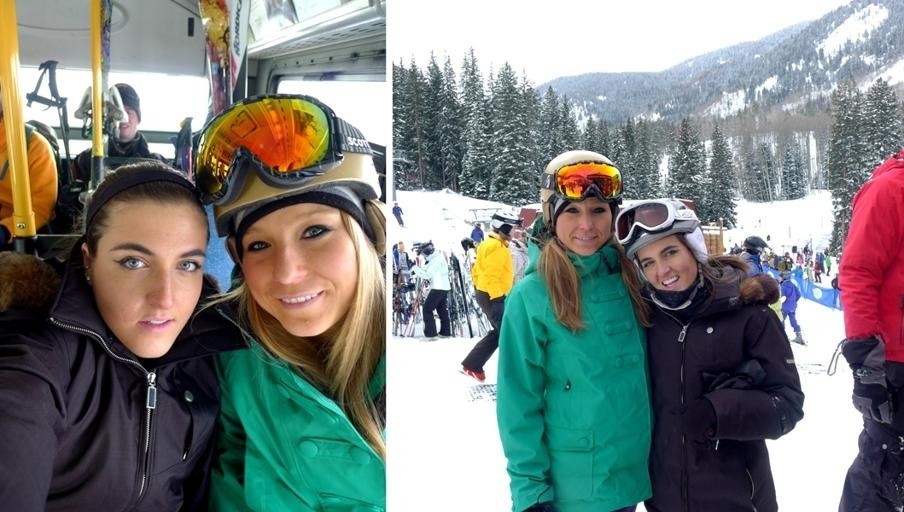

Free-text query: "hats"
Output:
<box><xmin>623</xmin><ymin>200</ymin><xmax>708</xmax><ymax>267</ymax></box>
<box><xmin>541</xmin><ymin>149</ymin><xmax>624</xmax><ymax>228</ymax></box>
<box><xmin>744</xmin><ymin>236</ymin><xmax>767</xmax><ymax>253</ymax></box>
<box><xmin>490</xmin><ymin>210</ymin><xmax>523</xmax><ymax>231</ymax></box>
<box><xmin>109</xmin><ymin>83</ymin><xmax>142</xmax><ymax>124</ymax></box>
<box><xmin>190</xmin><ymin>92</ymin><xmax>382</xmax><ymax>239</ymax></box>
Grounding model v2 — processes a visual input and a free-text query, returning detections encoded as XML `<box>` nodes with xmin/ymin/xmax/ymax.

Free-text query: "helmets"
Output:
<box><xmin>416</xmin><ymin>239</ymin><xmax>435</xmax><ymax>254</ymax></box>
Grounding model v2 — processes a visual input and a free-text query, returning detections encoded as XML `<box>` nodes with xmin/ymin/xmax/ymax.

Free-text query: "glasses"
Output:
<box><xmin>195</xmin><ymin>92</ymin><xmax>344</xmax><ymax>206</ymax></box>
<box><xmin>542</xmin><ymin>160</ymin><xmax>623</xmax><ymax>202</ymax></box>
<box><xmin>615</xmin><ymin>200</ymin><xmax>698</xmax><ymax>246</ymax></box>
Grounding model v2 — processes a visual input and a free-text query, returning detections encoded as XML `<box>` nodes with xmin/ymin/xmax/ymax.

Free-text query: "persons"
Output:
<box><xmin>0</xmin><ymin>161</ymin><xmax>249</xmax><ymax>512</ymax></box>
<box><xmin>461</xmin><ymin>237</ymin><xmax>482</xmax><ymax>324</ymax></box>
<box><xmin>614</xmin><ymin>196</ymin><xmax>804</xmax><ymax>512</ymax></box>
<box><xmin>761</xmin><ymin>244</ymin><xmax>770</xmax><ymax>267</ymax></box>
<box><xmin>496</xmin><ymin>150</ymin><xmax>654</xmax><ymax>511</ymax></box>
<box><xmin>392</xmin><ymin>203</ymin><xmax>404</xmax><ymax>227</ymax></box>
<box><xmin>191</xmin><ymin>91</ymin><xmax>386</xmax><ymax>511</ymax></box>
<box><xmin>459</xmin><ymin>209</ymin><xmax>521</xmax><ymax>382</ymax></box>
<box><xmin>770</xmin><ymin>238</ymin><xmax>841</xmax><ymax>282</ymax></box>
<box><xmin>393</xmin><ymin>242</ymin><xmax>413</xmax><ymax>325</ymax></box>
<box><xmin>739</xmin><ymin>236</ymin><xmax>767</xmax><ymax>276</ymax></box>
<box><xmin>56</xmin><ymin>83</ymin><xmax>183</xmax><ymax>233</ymax></box>
<box><xmin>837</xmin><ymin>147</ymin><xmax>904</xmax><ymax>510</ymax></box>
<box><xmin>471</xmin><ymin>223</ymin><xmax>484</xmax><ymax>243</ymax></box>
<box><xmin>508</xmin><ymin>226</ymin><xmax>528</xmax><ymax>285</ymax></box>
<box><xmin>777</xmin><ymin>274</ymin><xmax>805</xmax><ymax>345</ymax></box>
<box><xmin>422</xmin><ymin>242</ymin><xmax>451</xmax><ymax>340</ymax></box>
<box><xmin>0</xmin><ymin>108</ymin><xmax>59</xmax><ymax>253</ymax></box>
<box><xmin>729</xmin><ymin>243</ymin><xmax>741</xmax><ymax>254</ymax></box>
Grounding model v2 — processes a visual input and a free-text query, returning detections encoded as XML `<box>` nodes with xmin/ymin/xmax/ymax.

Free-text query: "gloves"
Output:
<box><xmin>669</xmin><ymin>398</ymin><xmax>713</xmax><ymax>439</ymax></box>
<box><xmin>841</xmin><ymin>335</ymin><xmax>893</xmax><ymax>424</ymax></box>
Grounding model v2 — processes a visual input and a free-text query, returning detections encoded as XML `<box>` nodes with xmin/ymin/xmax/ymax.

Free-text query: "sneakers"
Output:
<box><xmin>459</xmin><ymin>365</ymin><xmax>486</xmax><ymax>382</ymax></box>
<box><xmin>794</xmin><ymin>331</ymin><xmax>802</xmax><ymax>342</ymax></box>
